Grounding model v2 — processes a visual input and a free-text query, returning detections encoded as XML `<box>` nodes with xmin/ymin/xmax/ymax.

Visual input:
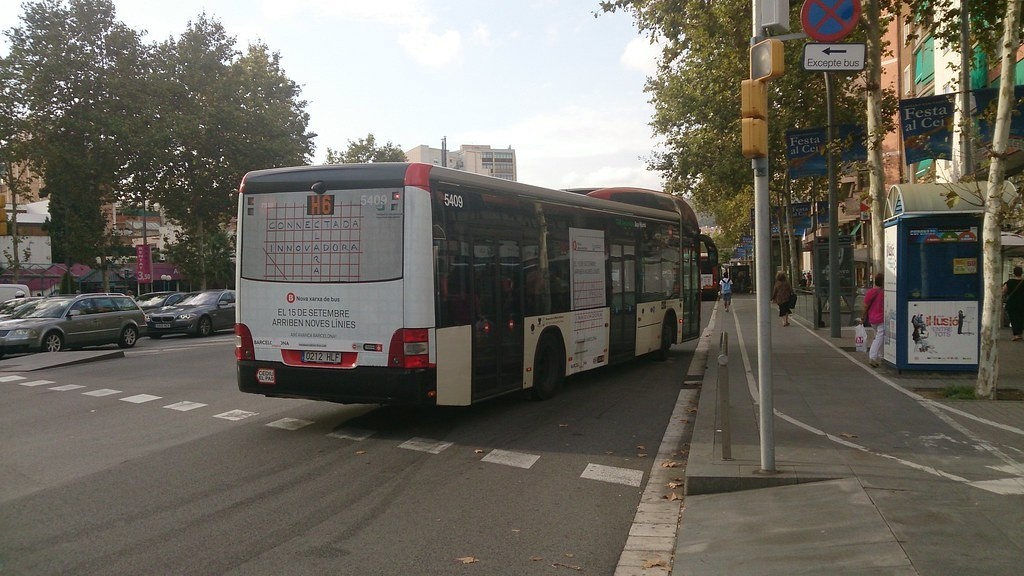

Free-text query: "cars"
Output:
<box><xmin>141</xmin><ymin>288</ymin><xmax>236</xmax><ymax>338</ymax></box>
<box><xmin>0</xmin><ymin>293</ymin><xmax>47</xmax><ymax>322</ymax></box>
<box><xmin>131</xmin><ymin>291</ymin><xmax>195</xmax><ymax>318</ymax></box>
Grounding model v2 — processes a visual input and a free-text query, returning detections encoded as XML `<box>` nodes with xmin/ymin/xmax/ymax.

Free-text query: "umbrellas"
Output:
<box><xmin>1001</xmin><ymin>231</ymin><xmax>1024</xmax><ymax>283</ymax></box>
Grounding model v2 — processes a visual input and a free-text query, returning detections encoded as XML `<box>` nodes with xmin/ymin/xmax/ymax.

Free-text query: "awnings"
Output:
<box><xmin>850</xmin><ymin>223</ymin><xmax>861</xmax><ymax>236</ymax></box>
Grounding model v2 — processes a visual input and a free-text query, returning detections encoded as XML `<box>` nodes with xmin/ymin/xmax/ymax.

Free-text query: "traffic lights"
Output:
<box><xmin>747</xmin><ymin>37</ymin><xmax>786</xmax><ymax>84</ymax></box>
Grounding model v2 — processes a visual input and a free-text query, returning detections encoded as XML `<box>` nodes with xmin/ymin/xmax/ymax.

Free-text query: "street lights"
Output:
<box><xmin>161</xmin><ymin>274</ymin><xmax>167</xmax><ymax>292</ymax></box>
<box><xmin>165</xmin><ymin>275</ymin><xmax>171</xmax><ymax>293</ymax></box>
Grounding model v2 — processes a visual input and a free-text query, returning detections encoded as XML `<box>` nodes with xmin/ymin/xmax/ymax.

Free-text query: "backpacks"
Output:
<box><xmin>722</xmin><ymin>278</ymin><xmax>731</xmax><ymax>295</ymax></box>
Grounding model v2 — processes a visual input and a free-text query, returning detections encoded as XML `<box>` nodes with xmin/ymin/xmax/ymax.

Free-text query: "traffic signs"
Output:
<box><xmin>803</xmin><ymin>42</ymin><xmax>869</xmax><ymax>71</ymax></box>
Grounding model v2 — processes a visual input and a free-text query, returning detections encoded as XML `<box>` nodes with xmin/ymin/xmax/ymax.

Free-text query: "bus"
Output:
<box><xmin>233</xmin><ymin>162</ymin><xmax>719</xmax><ymax>411</ymax></box>
<box><xmin>700</xmin><ymin>252</ymin><xmax>722</xmax><ymax>301</ymax></box>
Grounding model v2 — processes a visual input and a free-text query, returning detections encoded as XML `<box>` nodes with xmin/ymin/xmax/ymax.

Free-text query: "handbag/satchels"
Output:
<box><xmin>790</xmin><ymin>291</ymin><xmax>797</xmax><ymax>308</ymax></box>
<box><xmin>863</xmin><ymin>312</ymin><xmax>867</xmax><ymax>326</ymax></box>
<box><xmin>855</xmin><ymin>324</ymin><xmax>867</xmax><ymax>352</ymax></box>
<box><xmin>1002</xmin><ymin>302</ymin><xmax>1007</xmax><ymax>309</ymax></box>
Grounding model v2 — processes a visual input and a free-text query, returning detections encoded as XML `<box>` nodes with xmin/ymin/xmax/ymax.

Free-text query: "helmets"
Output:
<box><xmin>16</xmin><ymin>291</ymin><xmax>25</xmax><ymax>298</ymax></box>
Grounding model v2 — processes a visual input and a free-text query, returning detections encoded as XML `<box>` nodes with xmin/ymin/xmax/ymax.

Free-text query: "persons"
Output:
<box><xmin>859</xmin><ymin>273</ymin><xmax>884</xmax><ymax>368</ymax></box>
<box><xmin>771</xmin><ymin>270</ymin><xmax>793</xmax><ymax>327</ymax></box>
<box><xmin>802</xmin><ymin>272</ymin><xmax>812</xmax><ymax>286</ymax></box>
<box><xmin>958</xmin><ymin>310</ymin><xmax>965</xmax><ymax>335</ymax></box>
<box><xmin>912</xmin><ymin>314</ymin><xmax>927</xmax><ymax>344</ymax></box>
<box><xmin>719</xmin><ymin>271</ymin><xmax>734</xmax><ymax>312</ymax></box>
<box><xmin>1002</xmin><ymin>267</ymin><xmax>1024</xmax><ymax>341</ymax></box>
<box><xmin>126</xmin><ymin>290</ymin><xmax>135</xmax><ymax>299</ymax></box>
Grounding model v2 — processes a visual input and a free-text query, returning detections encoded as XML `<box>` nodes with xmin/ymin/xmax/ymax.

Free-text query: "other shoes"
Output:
<box><xmin>1011</xmin><ymin>334</ymin><xmax>1022</xmax><ymax>340</ymax></box>
<box><xmin>725</xmin><ymin>308</ymin><xmax>729</xmax><ymax>312</ymax></box>
<box><xmin>784</xmin><ymin>322</ymin><xmax>790</xmax><ymax>327</ymax></box>
<box><xmin>868</xmin><ymin>359</ymin><xmax>878</xmax><ymax>368</ymax></box>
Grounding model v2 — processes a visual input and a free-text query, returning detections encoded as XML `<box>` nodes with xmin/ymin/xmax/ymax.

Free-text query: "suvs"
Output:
<box><xmin>0</xmin><ymin>292</ymin><xmax>149</xmax><ymax>354</ymax></box>
